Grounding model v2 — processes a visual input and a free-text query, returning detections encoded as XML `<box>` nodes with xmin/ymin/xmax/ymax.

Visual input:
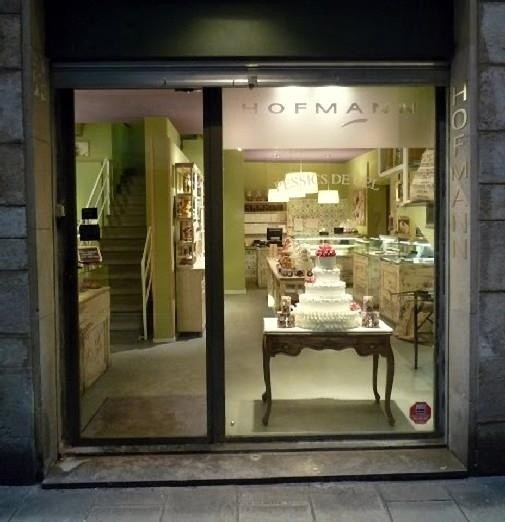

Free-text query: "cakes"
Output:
<box><xmin>291</xmin><ymin>244</ymin><xmax>360</xmax><ymax>331</ymax></box>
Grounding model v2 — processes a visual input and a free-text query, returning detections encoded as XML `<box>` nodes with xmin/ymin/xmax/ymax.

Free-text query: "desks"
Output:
<box><xmin>266</xmin><ymin>256</ymin><xmax>339</xmax><ymax>314</ymax></box>
<box><xmin>263</xmin><ymin>317</ymin><xmax>397</xmax><ymax>428</ymax></box>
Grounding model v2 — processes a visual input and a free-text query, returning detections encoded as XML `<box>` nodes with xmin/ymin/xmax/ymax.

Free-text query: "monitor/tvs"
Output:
<box><xmin>267</xmin><ymin>228</ymin><xmax>282</xmax><ymax>244</ymax></box>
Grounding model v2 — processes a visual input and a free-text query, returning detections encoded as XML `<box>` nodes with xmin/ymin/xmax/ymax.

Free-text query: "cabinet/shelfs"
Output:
<box><xmin>176</xmin><ymin>269</ymin><xmax>205</xmax><ymax>336</ymax></box>
<box><xmin>243</xmin><ymin>202</ymin><xmax>289</xmax><ymax>235</ymax></box>
<box><xmin>378</xmin><ymin>148</ymin><xmax>434</xmax><ymax>207</ymax></box>
<box><xmin>351</xmin><ymin>252</ymin><xmax>382</xmax><ymax>309</ymax></box>
<box><xmin>257</xmin><ymin>233</ymin><xmax>359</xmax><ymax>288</ymax></box>
<box><xmin>175</xmin><ymin>162</ymin><xmax>205</xmax><ymax>336</ymax></box>
<box><xmin>79</xmin><ymin>287</ymin><xmax>112</xmax><ymax>393</ymax></box>
<box><xmin>379</xmin><ymin>258</ymin><xmax>435</xmax><ymax>342</ymax></box>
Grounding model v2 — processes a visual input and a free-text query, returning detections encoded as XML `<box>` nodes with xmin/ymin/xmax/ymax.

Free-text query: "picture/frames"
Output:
<box><xmin>75</xmin><ymin>123</ymin><xmax>89</xmax><ymax>156</ymax></box>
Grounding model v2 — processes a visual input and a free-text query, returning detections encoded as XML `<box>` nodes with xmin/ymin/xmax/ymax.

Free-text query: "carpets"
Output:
<box><xmin>79</xmin><ymin>396</ymin><xmax>207</xmax><ymax>438</ymax></box>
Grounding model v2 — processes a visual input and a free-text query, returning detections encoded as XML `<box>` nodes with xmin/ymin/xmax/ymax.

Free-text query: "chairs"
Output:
<box><xmin>80</xmin><ymin>207</ymin><xmax>103</xmax><ymax>242</ymax></box>
<box><xmin>79</xmin><ymin>244</ymin><xmax>101</xmax><ymax>277</ymax></box>
<box><xmin>414</xmin><ymin>289</ymin><xmax>434</xmax><ymax>370</ymax></box>
